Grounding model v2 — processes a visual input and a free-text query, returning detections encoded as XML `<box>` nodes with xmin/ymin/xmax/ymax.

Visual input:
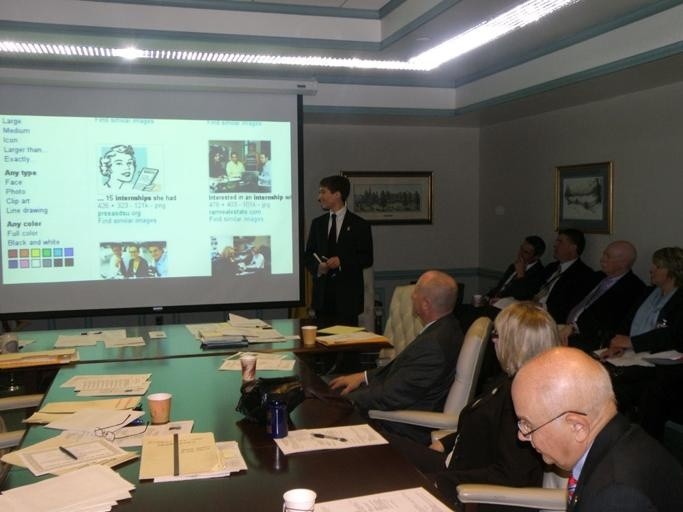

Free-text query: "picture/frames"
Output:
<box><xmin>341</xmin><ymin>169</ymin><xmax>434</xmax><ymax>227</ymax></box>
<box><xmin>551</xmin><ymin>159</ymin><xmax>614</xmax><ymax>235</ymax></box>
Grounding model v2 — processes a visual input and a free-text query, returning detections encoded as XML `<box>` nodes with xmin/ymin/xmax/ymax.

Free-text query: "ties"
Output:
<box><xmin>327</xmin><ymin>214</ymin><xmax>341</xmax><ymax>280</ymax></box>
<box><xmin>563</xmin><ymin>475</ymin><xmax>578</xmax><ymax>509</ymax></box>
<box><xmin>531</xmin><ymin>269</ymin><xmax>562</xmax><ymax>307</ymax></box>
<box><xmin>565</xmin><ymin>279</ymin><xmax>611</xmax><ymax>327</ymax></box>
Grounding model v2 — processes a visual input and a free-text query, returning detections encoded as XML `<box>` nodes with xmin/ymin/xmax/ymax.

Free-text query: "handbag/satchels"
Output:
<box><xmin>235</xmin><ymin>374</ymin><xmax>355</xmax><ymax>422</ymax></box>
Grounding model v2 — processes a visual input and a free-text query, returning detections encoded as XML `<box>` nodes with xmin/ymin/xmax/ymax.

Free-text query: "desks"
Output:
<box><xmin>2</xmin><ymin>319</ymin><xmax>392</xmax><ymax>361</ymax></box>
<box><xmin>1</xmin><ymin>354</ymin><xmax>462</xmax><ymax>512</ymax></box>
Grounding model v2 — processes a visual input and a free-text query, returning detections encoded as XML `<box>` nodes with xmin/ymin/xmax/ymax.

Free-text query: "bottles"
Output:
<box><xmin>269</xmin><ymin>399</ymin><xmax>288</xmax><ymax>439</ymax></box>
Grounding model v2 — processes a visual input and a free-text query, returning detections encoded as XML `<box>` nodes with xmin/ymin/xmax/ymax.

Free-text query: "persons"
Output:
<box><xmin>304</xmin><ymin>175</ymin><xmax>373</xmax><ymax>326</ymax></box>
<box><xmin>126</xmin><ymin>246</ymin><xmax>148</xmax><ymax>276</ymax></box>
<box><xmin>98</xmin><ymin>144</ymin><xmax>156</xmax><ymax>191</ymax></box>
<box><xmin>589</xmin><ymin>178</ymin><xmax>601</xmax><ymax>204</ymax></box>
<box><xmin>565</xmin><ymin>183</ymin><xmax>574</xmax><ymax>205</ymax></box>
<box><xmin>510</xmin><ymin>346</ymin><xmax>682</xmax><ymax>511</ymax></box>
<box><xmin>352</xmin><ymin>189</ymin><xmax>420</xmax><ymax>211</ymax></box>
<box><xmin>110</xmin><ymin>244</ymin><xmax>126</xmax><ymax>278</ymax></box>
<box><xmin>544</xmin><ymin>228</ymin><xmax>598</xmax><ymax>323</ymax></box>
<box><xmin>208</xmin><ymin>237</ymin><xmax>266</xmax><ymax>276</ymax></box>
<box><xmin>148</xmin><ymin>243</ymin><xmax>167</xmax><ymax>276</ymax></box>
<box><xmin>600</xmin><ymin>248</ymin><xmax>681</xmax><ymax>360</ymax></box>
<box><xmin>484</xmin><ymin>235</ymin><xmax>547</xmax><ymax>298</ymax></box>
<box><xmin>208</xmin><ymin>142</ymin><xmax>271</xmax><ymax>191</ymax></box>
<box><xmin>558</xmin><ymin>240</ymin><xmax>649</xmax><ymax>347</ymax></box>
<box><xmin>320</xmin><ymin>269</ymin><xmax>461</xmax><ymax>446</ymax></box>
<box><xmin>426</xmin><ymin>299</ymin><xmax>560</xmax><ymax>487</ymax></box>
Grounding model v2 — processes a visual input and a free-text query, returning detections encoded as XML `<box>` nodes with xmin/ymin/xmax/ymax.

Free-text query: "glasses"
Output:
<box><xmin>489</xmin><ymin>326</ymin><xmax>498</xmax><ymax>339</ymax></box>
<box><xmin>516</xmin><ymin>410</ymin><xmax>590</xmax><ymax>438</ymax></box>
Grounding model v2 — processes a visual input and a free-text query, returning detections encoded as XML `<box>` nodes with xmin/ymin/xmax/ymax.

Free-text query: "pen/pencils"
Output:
<box><xmin>314</xmin><ymin>434</ymin><xmax>347</xmax><ymax>442</ymax></box>
<box><xmin>600</xmin><ymin>351</ymin><xmax>621</xmax><ymax>362</ymax></box>
<box><xmin>81</xmin><ymin>332</ymin><xmax>102</xmax><ymax>335</ymax></box>
<box><xmin>59</xmin><ymin>447</ymin><xmax>77</xmax><ymax>459</ymax></box>
<box><xmin>313</xmin><ymin>253</ymin><xmax>322</xmax><ymax>263</ymax></box>
<box><xmin>18</xmin><ymin>341</ymin><xmax>34</xmax><ymax>349</ymax></box>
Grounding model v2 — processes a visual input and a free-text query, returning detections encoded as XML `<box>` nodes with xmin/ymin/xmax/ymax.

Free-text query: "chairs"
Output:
<box><xmin>370</xmin><ymin>313</ymin><xmax>496</xmax><ymax>435</ymax></box>
<box><xmin>376</xmin><ymin>284</ymin><xmax>435</xmax><ymax>370</ymax></box>
<box><xmin>0</xmin><ymin>391</ymin><xmax>43</xmax><ymax>482</ymax></box>
<box><xmin>459</xmin><ymin>470</ymin><xmax>572</xmax><ymax>508</ymax></box>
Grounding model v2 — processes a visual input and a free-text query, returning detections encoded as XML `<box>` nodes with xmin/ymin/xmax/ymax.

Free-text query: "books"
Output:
<box><xmin>137</xmin><ymin>431</ymin><xmax>247</xmax><ymax>482</ymax></box>
<box><xmin>185</xmin><ymin>313</ymin><xmax>289</xmax><ymax>350</ymax></box>
<box><xmin>0</xmin><ymin>349</ymin><xmax>80</xmax><ymax>369</ymax></box>
<box><xmin>20</xmin><ymin>394</ymin><xmax>142</xmax><ymax>426</ymax></box>
<box><xmin>318</xmin><ymin>324</ymin><xmax>391</xmax><ymax>346</ymax></box>
<box><xmin>593</xmin><ymin>343</ymin><xmax>656</xmax><ymax>370</ymax></box>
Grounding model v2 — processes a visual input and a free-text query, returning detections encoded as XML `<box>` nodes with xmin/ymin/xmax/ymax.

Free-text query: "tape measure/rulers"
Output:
<box><xmin>174</xmin><ymin>434</ymin><xmax>179</xmax><ymax>476</ymax></box>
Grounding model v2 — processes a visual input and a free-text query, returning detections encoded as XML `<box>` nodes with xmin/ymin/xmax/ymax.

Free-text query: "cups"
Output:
<box><xmin>0</xmin><ymin>335</ymin><xmax>18</xmax><ymax>355</ymax></box>
<box><xmin>240</xmin><ymin>355</ymin><xmax>257</xmax><ymax>382</ymax></box>
<box><xmin>472</xmin><ymin>294</ymin><xmax>481</xmax><ymax>308</ymax></box>
<box><xmin>281</xmin><ymin>486</ymin><xmax>317</xmax><ymax>512</ymax></box>
<box><xmin>301</xmin><ymin>325</ymin><xmax>317</xmax><ymax>346</ymax></box>
<box><xmin>147</xmin><ymin>393</ymin><xmax>171</xmax><ymax>425</ymax></box>
